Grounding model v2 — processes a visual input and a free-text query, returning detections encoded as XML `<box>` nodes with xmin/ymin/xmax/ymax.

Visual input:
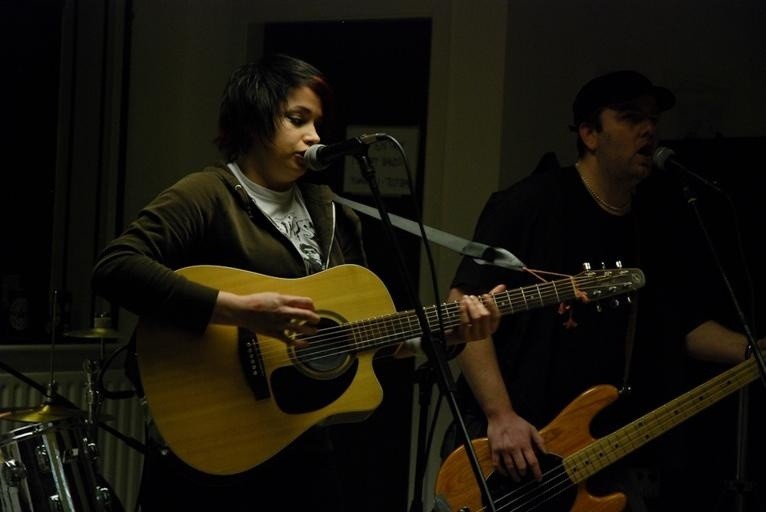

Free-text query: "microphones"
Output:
<box><xmin>652</xmin><ymin>147</ymin><xmax>728</xmax><ymax>200</ymax></box>
<box><xmin>303</xmin><ymin>132</ymin><xmax>388</xmax><ymax>170</ymax></box>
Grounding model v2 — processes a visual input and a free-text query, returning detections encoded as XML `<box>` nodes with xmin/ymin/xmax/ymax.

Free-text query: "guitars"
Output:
<box><xmin>135</xmin><ymin>262</ymin><xmax>645</xmax><ymax>473</ymax></box>
<box><xmin>433</xmin><ymin>349</ymin><xmax>766</xmax><ymax>511</ymax></box>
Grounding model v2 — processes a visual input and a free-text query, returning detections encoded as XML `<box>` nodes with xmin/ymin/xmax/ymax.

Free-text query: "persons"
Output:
<box><xmin>88</xmin><ymin>51</ymin><xmax>508</xmax><ymax>512</ymax></box>
<box><xmin>442</xmin><ymin>68</ymin><xmax>765</xmax><ymax>511</ymax></box>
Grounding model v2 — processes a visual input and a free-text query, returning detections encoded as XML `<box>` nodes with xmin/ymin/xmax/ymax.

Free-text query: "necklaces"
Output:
<box><xmin>570</xmin><ymin>160</ymin><xmax>638</xmax><ymax>219</ymax></box>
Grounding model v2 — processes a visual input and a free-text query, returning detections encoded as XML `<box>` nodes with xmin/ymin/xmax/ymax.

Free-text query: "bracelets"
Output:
<box><xmin>733</xmin><ymin>328</ymin><xmax>760</xmax><ymax>375</ymax></box>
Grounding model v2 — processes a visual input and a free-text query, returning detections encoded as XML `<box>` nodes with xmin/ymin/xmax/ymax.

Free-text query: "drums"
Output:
<box><xmin>0</xmin><ymin>420</ymin><xmax>113</xmax><ymax>512</ymax></box>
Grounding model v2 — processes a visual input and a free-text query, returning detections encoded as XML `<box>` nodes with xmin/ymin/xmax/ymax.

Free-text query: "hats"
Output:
<box><xmin>574</xmin><ymin>74</ymin><xmax>681</xmax><ymax>124</ymax></box>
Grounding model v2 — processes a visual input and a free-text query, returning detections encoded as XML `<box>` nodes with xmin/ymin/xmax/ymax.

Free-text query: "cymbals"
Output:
<box><xmin>0</xmin><ymin>405</ymin><xmax>117</xmax><ymax>423</ymax></box>
<box><xmin>62</xmin><ymin>328</ymin><xmax>130</xmax><ymax>338</ymax></box>
